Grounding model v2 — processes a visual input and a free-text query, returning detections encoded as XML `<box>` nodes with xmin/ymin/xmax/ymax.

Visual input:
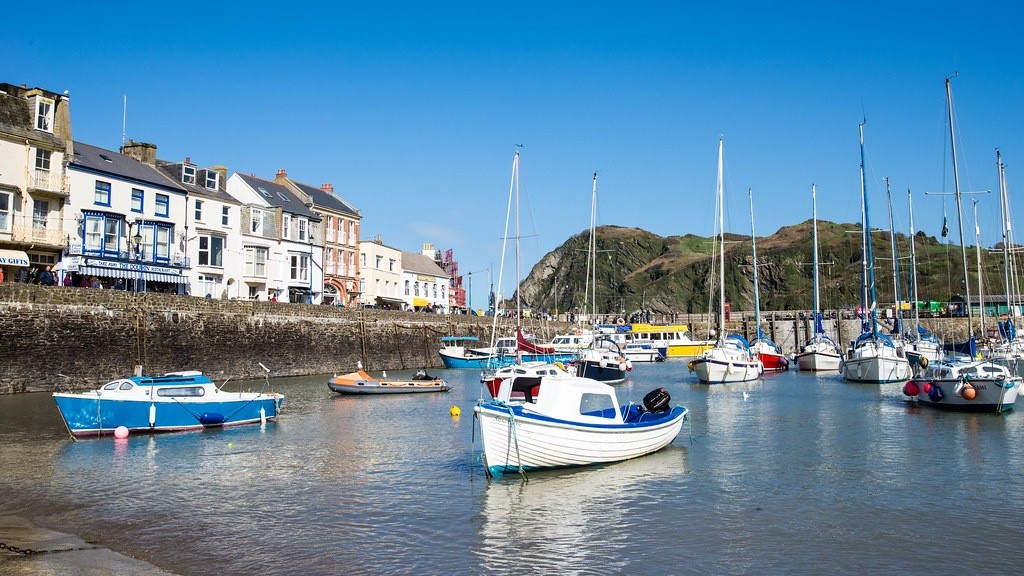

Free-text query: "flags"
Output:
<box><xmin>941</xmin><ymin>215</ymin><xmax>949</xmax><ymax>238</ymax></box>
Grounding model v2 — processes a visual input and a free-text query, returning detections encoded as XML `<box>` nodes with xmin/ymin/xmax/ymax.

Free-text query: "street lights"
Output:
<box><xmin>469</xmin><ymin>272</ymin><xmax>471</xmax><ymax>306</ymax></box>
<box><xmin>309</xmin><ymin>235</ymin><xmax>314</xmax><ymax>304</ymax></box>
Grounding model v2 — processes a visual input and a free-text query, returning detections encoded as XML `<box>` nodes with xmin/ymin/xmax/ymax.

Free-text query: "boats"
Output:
<box><xmin>468</xmin><ymin>323</ymin><xmax>739</xmax><ymax>362</ymax></box>
<box><xmin>327</xmin><ymin>370</ymin><xmax>451</xmax><ymax>393</ymax></box>
<box><xmin>50</xmin><ymin>371</ymin><xmax>285</xmax><ymax>434</ymax></box>
<box><xmin>438</xmin><ymin>336</ymin><xmax>499</xmax><ymax>369</ymax></box>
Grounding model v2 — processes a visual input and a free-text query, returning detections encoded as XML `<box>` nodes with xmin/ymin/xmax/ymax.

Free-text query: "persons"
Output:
<box><xmin>272</xmin><ymin>294</ymin><xmax>276</xmax><ymax>302</ymax></box>
<box><xmin>0</xmin><ymin>268</ymin><xmax>3</xmax><ymax>284</ymax></box>
<box><xmin>254</xmin><ymin>294</ymin><xmax>260</xmax><ymax>301</ymax></box>
<box><xmin>367</xmin><ymin>302</ymin><xmax>444</xmax><ymax>314</ymax></box>
<box><xmin>29</xmin><ymin>265</ymin><xmax>177</xmax><ymax>295</ymax></box>
<box><xmin>221</xmin><ymin>289</ymin><xmax>227</xmax><ymax>300</ymax></box>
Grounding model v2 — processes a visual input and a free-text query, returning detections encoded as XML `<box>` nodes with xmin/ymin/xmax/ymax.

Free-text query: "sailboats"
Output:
<box><xmin>748</xmin><ymin>187</ymin><xmax>790</xmax><ymax>370</ymax></box>
<box><xmin>795</xmin><ymin>184</ymin><xmax>846</xmax><ymax>371</ymax></box>
<box><xmin>575</xmin><ymin>172</ymin><xmax>626</xmax><ymax>383</ymax></box>
<box><xmin>909</xmin><ymin>77</ymin><xmax>1023</xmax><ymax>414</ymax></box>
<box><xmin>692</xmin><ymin>140</ymin><xmax>763</xmax><ymax>384</ymax></box>
<box><xmin>841</xmin><ymin>120</ymin><xmax>944</xmax><ymax>385</ymax></box>
<box><xmin>473</xmin><ymin>150</ymin><xmax>689</xmax><ymax>477</ymax></box>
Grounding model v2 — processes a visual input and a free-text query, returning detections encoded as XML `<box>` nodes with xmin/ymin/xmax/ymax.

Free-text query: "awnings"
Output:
<box><xmin>413</xmin><ymin>298</ymin><xmax>428</xmax><ymax>306</ymax></box>
<box><xmin>288</xmin><ymin>286</ymin><xmax>315</xmax><ymax>295</ymax></box>
<box><xmin>377</xmin><ymin>295</ymin><xmax>407</xmax><ymax>303</ymax></box>
<box><xmin>0</xmin><ymin>249</ymin><xmax>30</xmax><ymax>267</ymax></box>
<box><xmin>76</xmin><ymin>267</ymin><xmax>189</xmax><ymax>285</ymax></box>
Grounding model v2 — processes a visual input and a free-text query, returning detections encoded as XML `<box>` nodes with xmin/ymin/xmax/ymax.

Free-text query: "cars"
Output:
<box><xmin>363</xmin><ymin>305</ymin><xmax>378</xmax><ymax>309</ymax></box>
